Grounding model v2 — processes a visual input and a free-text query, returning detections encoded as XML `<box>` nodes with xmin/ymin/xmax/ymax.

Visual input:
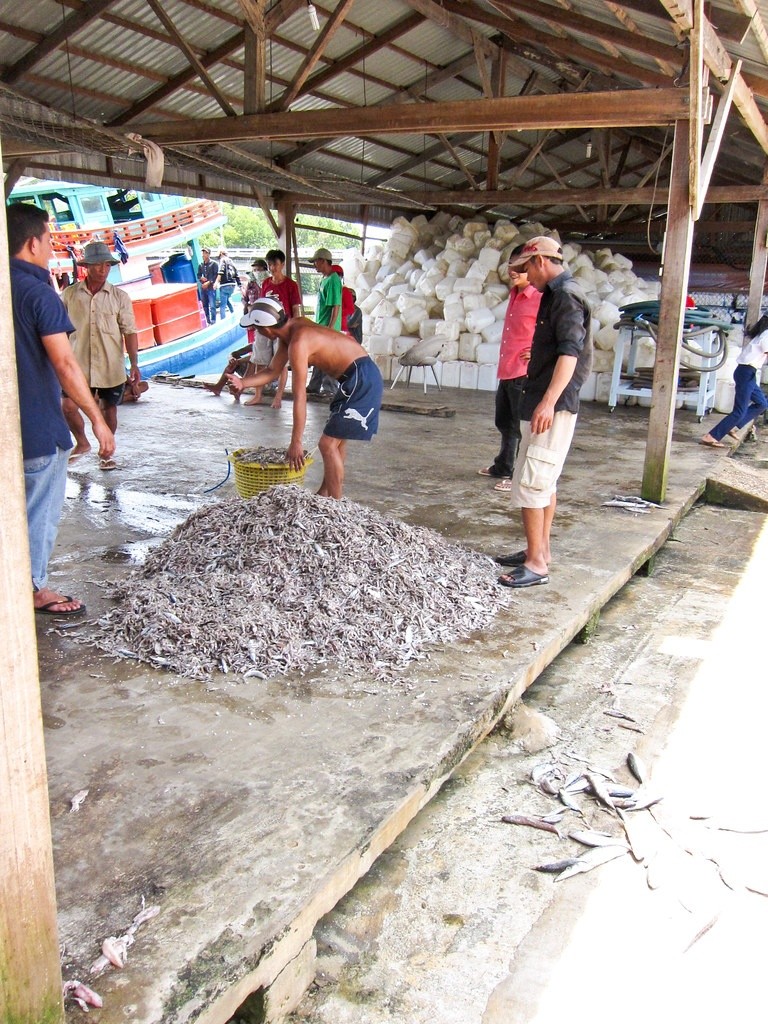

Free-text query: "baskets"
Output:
<box><xmin>226</xmin><ymin>449</ymin><xmax>314</xmax><ymax>500</ymax></box>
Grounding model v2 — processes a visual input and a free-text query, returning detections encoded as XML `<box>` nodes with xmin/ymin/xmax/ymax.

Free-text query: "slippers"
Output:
<box><xmin>68</xmin><ymin>448</ymin><xmax>91</xmax><ymax>468</ymax></box>
<box><xmin>99</xmin><ymin>456</ymin><xmax>116</xmax><ymax>469</ymax></box>
<box><xmin>728</xmin><ymin>430</ymin><xmax>740</xmax><ymax>440</ymax></box>
<box><xmin>494</xmin><ymin>479</ymin><xmax>513</xmax><ymax>491</ymax></box>
<box><xmin>477</xmin><ymin>465</ymin><xmax>510</xmax><ymax>479</ymax></box>
<box><xmin>498</xmin><ymin>564</ymin><xmax>550</xmax><ymax>587</ymax></box>
<box><xmin>698</xmin><ymin>435</ymin><xmax>726</xmax><ymax>448</ymax></box>
<box><xmin>495</xmin><ymin>551</ymin><xmax>550</xmax><ymax>569</ymax></box>
<box><xmin>34</xmin><ymin>595</ymin><xmax>86</xmax><ymax>615</ymax></box>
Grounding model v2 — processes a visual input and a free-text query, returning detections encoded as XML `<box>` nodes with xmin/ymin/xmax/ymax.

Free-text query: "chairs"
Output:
<box><xmin>389</xmin><ymin>340</ymin><xmax>444</xmax><ymax>395</ymax></box>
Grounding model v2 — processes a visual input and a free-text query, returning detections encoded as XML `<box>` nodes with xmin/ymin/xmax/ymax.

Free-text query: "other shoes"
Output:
<box><xmin>306</xmin><ymin>390</ymin><xmax>320</xmax><ymax>395</ymax></box>
<box><xmin>316</xmin><ymin>390</ymin><xmax>336</xmax><ymax>397</ymax></box>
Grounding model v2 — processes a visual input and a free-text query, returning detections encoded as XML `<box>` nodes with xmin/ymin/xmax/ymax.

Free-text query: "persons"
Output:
<box><xmin>203</xmin><ymin>339</ymin><xmax>280</xmax><ymax>399</ymax></box>
<box><xmin>5</xmin><ymin>202</ymin><xmax>116</xmax><ymax>616</ymax></box>
<box><xmin>197</xmin><ymin>247</ymin><xmax>219</xmax><ymax>325</ymax></box>
<box><xmin>244</xmin><ymin>250</ymin><xmax>301</xmax><ymax>409</ymax></box>
<box><xmin>213</xmin><ymin>250</ymin><xmax>245</xmax><ymax>320</ymax></box>
<box><xmin>59</xmin><ymin>242</ymin><xmax>149</xmax><ymax>471</ymax></box>
<box><xmin>331</xmin><ymin>264</ymin><xmax>354</xmax><ymax>336</ymax></box>
<box><xmin>477</xmin><ymin>244</ymin><xmax>543</xmax><ymax>492</ymax></box>
<box><xmin>242</xmin><ymin>259</ymin><xmax>269</xmax><ymax>344</ymax></box>
<box><xmin>699</xmin><ymin>308</ymin><xmax>768</xmax><ymax>447</ymax></box>
<box><xmin>305</xmin><ymin>248</ymin><xmax>343</xmax><ymax>396</ymax></box>
<box><xmin>497</xmin><ymin>236</ymin><xmax>592</xmax><ymax>588</ymax></box>
<box><xmin>348</xmin><ymin>288</ymin><xmax>362</xmax><ymax>346</ymax></box>
<box><xmin>224</xmin><ymin>297</ymin><xmax>384</xmax><ymax>497</ymax></box>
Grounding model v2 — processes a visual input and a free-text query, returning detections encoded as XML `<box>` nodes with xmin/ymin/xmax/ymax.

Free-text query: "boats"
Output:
<box><xmin>6</xmin><ymin>181</ymin><xmax>249</xmax><ymax>379</ymax></box>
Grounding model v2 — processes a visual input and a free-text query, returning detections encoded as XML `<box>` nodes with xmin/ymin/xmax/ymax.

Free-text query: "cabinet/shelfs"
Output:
<box><xmin>607</xmin><ymin>329</ymin><xmax>720</xmax><ymax>422</ymax></box>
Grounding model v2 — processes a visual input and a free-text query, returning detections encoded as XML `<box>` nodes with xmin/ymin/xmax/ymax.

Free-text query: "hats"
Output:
<box><xmin>239</xmin><ymin>297</ymin><xmax>285</xmax><ymax>328</ymax></box>
<box><xmin>507</xmin><ymin>235</ymin><xmax>563</xmax><ymax>267</ymax></box>
<box><xmin>251</xmin><ymin>259</ymin><xmax>267</xmax><ymax>268</ymax></box>
<box><xmin>218</xmin><ymin>249</ymin><xmax>228</xmax><ymax>256</ymax></box>
<box><xmin>201</xmin><ymin>248</ymin><xmax>211</xmax><ymax>254</ymax></box>
<box><xmin>349</xmin><ymin>288</ymin><xmax>356</xmax><ymax>296</ymax></box>
<box><xmin>307</xmin><ymin>248</ymin><xmax>332</xmax><ymax>263</ymax></box>
<box><xmin>331</xmin><ymin>265</ymin><xmax>342</xmax><ymax>273</ymax></box>
<box><xmin>77</xmin><ymin>241</ymin><xmax>121</xmax><ymax>266</ymax></box>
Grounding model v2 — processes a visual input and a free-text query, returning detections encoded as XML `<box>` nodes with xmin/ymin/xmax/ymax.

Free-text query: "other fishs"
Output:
<box><xmin>500</xmin><ymin>708</ymin><xmax>768</xmax><ymax>954</ymax></box>
<box><xmin>46</xmin><ymin>446</ymin><xmax>513</xmax><ymax>698</ymax></box>
<box><xmin>603</xmin><ymin>493</ymin><xmax>669</xmax><ymax>513</ymax></box>
